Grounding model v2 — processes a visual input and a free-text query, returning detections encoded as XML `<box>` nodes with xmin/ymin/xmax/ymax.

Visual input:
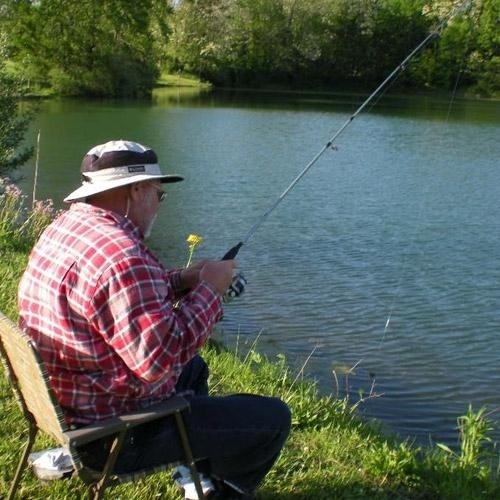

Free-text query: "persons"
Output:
<box><xmin>13</xmin><ymin>139</ymin><xmax>293</xmax><ymax>498</ymax></box>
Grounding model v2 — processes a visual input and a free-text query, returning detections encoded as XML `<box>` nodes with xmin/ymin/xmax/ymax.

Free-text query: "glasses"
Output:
<box><xmin>149</xmin><ymin>182</ymin><xmax>167</xmax><ymax>201</ymax></box>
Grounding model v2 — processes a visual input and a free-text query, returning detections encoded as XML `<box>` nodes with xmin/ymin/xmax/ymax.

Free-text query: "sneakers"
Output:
<box><xmin>173</xmin><ymin>466</ymin><xmax>217</xmax><ymax>499</ymax></box>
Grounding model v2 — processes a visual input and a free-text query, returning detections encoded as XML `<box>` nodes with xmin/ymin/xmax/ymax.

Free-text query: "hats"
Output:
<box><xmin>63</xmin><ymin>140</ymin><xmax>185</xmax><ymax>204</ymax></box>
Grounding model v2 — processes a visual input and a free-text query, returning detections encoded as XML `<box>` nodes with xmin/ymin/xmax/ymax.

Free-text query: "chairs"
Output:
<box><xmin>0</xmin><ymin>311</ymin><xmax>205</xmax><ymax>500</ymax></box>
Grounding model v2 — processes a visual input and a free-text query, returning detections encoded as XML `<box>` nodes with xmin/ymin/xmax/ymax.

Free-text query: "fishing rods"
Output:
<box><xmin>221</xmin><ymin>1</ymin><xmax>471</xmax><ymax>261</ymax></box>
